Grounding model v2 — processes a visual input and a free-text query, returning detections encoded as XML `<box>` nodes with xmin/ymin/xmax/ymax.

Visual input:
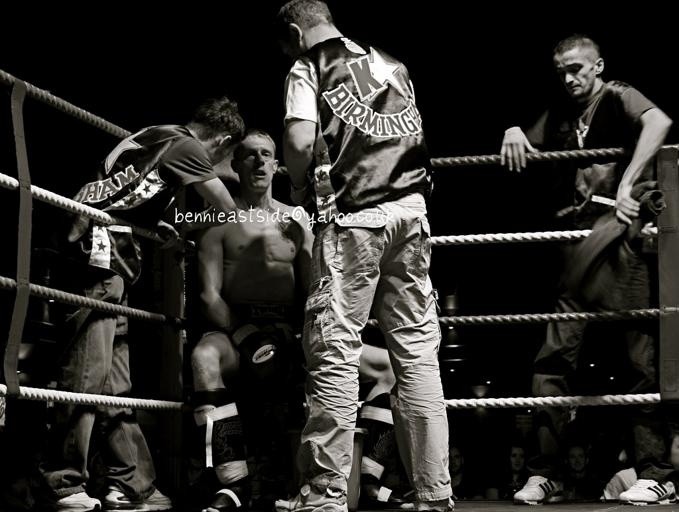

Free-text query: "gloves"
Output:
<box><xmin>232</xmin><ymin>320</ymin><xmax>299</xmax><ymax>371</ymax></box>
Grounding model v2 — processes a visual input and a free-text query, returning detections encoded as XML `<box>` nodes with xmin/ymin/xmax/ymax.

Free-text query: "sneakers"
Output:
<box><xmin>396</xmin><ymin>493</ymin><xmax>456</xmax><ymax>511</ymax></box>
<box><xmin>272</xmin><ymin>487</ymin><xmax>348</xmax><ymax>511</ymax></box>
<box><xmin>618</xmin><ymin>476</ymin><xmax>678</xmax><ymax>508</ymax></box>
<box><xmin>55</xmin><ymin>491</ymin><xmax>104</xmax><ymax>512</ymax></box>
<box><xmin>104</xmin><ymin>484</ymin><xmax>173</xmax><ymax>511</ymax></box>
<box><xmin>512</xmin><ymin>475</ymin><xmax>571</xmax><ymax>505</ymax></box>
